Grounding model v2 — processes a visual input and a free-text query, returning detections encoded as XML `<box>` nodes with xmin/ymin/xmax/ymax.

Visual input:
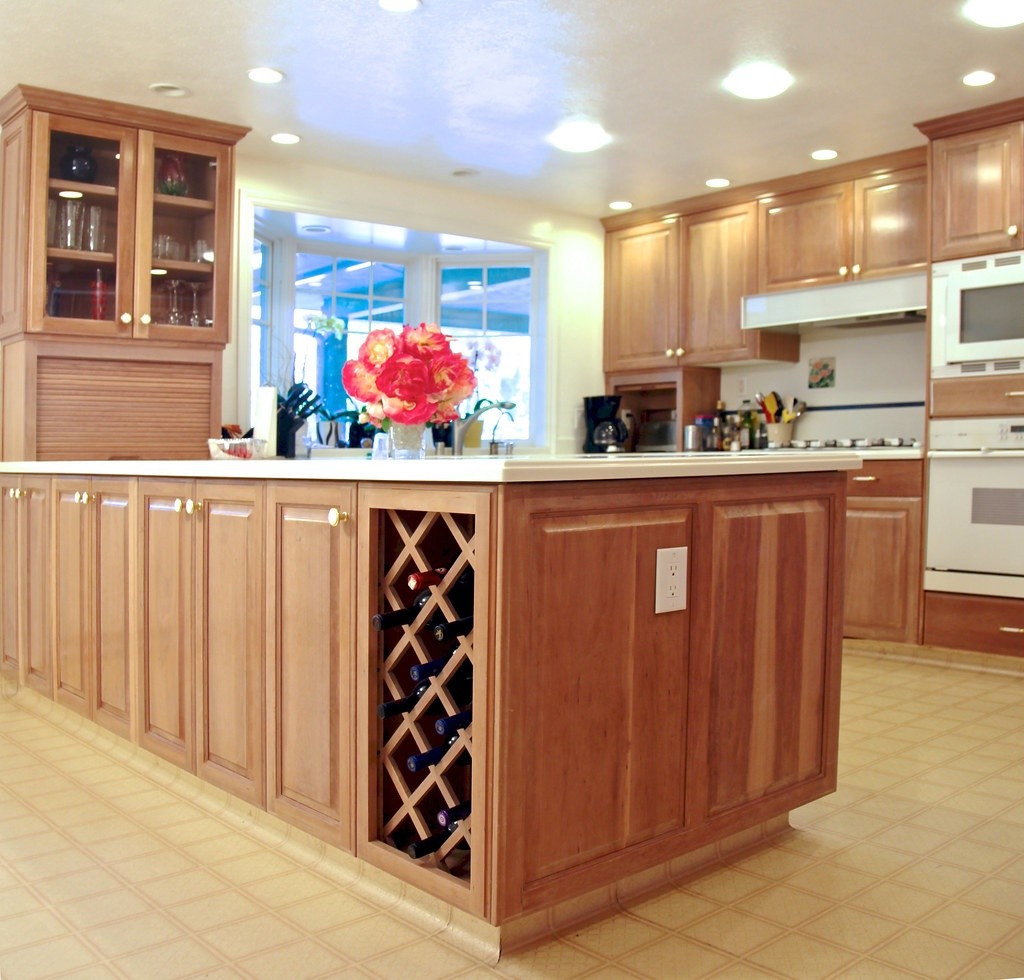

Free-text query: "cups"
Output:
<box><xmin>765</xmin><ymin>423</ymin><xmax>793</xmax><ymax>445</ymax></box>
<box><xmin>46</xmin><ymin>198</ymin><xmax>108</xmax><ymax>253</ymax></box>
<box><xmin>90</xmin><ymin>268</ymin><xmax>108</xmax><ymax>320</ymax></box>
<box><xmin>152</xmin><ymin>231</ymin><xmax>208</xmax><ymax>263</ymax></box>
<box><xmin>683</xmin><ymin>424</ymin><xmax>702</xmax><ymax>451</ymax></box>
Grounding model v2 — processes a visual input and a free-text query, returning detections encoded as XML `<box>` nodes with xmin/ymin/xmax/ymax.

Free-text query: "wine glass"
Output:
<box><xmin>157</xmin><ymin>278</ymin><xmax>206</xmax><ymax>326</ymax></box>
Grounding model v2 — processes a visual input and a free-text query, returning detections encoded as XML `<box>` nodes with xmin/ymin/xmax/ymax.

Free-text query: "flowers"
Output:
<box><xmin>341</xmin><ymin>323</ymin><xmax>478</xmax><ymax>424</ymax></box>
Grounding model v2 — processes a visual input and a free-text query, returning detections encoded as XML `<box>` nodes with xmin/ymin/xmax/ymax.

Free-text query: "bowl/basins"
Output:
<box><xmin>207</xmin><ymin>438</ymin><xmax>266</xmax><ymax>460</ymax></box>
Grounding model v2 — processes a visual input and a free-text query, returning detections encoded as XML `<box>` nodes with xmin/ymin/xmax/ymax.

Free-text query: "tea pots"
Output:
<box><xmin>593</xmin><ymin>417</ymin><xmax>628</xmax><ymax>446</ymax></box>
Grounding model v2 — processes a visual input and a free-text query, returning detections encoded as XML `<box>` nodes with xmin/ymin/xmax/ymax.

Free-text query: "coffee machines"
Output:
<box><xmin>581</xmin><ymin>394</ymin><xmax>628</xmax><ymax>453</ymax></box>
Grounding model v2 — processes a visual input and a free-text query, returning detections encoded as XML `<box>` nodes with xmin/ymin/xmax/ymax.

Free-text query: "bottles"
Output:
<box><xmin>372</xmin><ymin>565</ymin><xmax>473</xmax><ymax>858</ymax></box>
<box><xmin>157</xmin><ymin>153</ymin><xmax>187</xmax><ymax>195</ymax></box>
<box><xmin>58</xmin><ymin>144</ymin><xmax>97</xmax><ymax>182</ymax></box>
<box><xmin>705</xmin><ymin>398</ymin><xmax>760</xmax><ymax>450</ymax></box>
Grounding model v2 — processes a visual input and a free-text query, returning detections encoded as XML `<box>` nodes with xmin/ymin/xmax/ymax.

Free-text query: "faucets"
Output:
<box><xmin>452</xmin><ymin>401</ymin><xmax>517</xmax><ymax>456</ymax></box>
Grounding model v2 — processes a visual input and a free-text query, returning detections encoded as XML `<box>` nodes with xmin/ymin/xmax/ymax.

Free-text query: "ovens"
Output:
<box><xmin>923</xmin><ymin>419</ymin><xmax>1024</xmax><ymax>598</ymax></box>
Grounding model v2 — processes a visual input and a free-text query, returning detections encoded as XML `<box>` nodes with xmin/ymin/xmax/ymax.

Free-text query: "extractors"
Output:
<box><xmin>739</xmin><ymin>271</ymin><xmax>928</xmax><ymax>334</ymax></box>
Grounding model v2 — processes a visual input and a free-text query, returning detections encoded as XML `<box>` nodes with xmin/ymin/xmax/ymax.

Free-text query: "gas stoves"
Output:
<box><xmin>766</xmin><ymin>435</ymin><xmax>923</xmax><ymax>459</ymax></box>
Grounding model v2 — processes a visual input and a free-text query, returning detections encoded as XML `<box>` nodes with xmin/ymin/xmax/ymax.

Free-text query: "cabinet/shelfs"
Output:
<box><xmin>0</xmin><ymin>473</ymin><xmax>356</xmax><ymax>856</ymax></box>
<box><xmin>0</xmin><ymin>84</ymin><xmax>253</xmax><ymax>352</ymax></box>
<box><xmin>844</xmin><ymin>456</ymin><xmax>926</xmax><ymax>643</ymax></box>
<box><xmin>356</xmin><ymin>482</ymin><xmax>492</xmax><ymax>920</ymax></box>
<box><xmin>498</xmin><ymin>471</ymin><xmax>865</xmax><ymax>929</ymax></box>
<box><xmin>600</xmin><ymin>97</ymin><xmax>1024</xmax><ymax>450</ymax></box>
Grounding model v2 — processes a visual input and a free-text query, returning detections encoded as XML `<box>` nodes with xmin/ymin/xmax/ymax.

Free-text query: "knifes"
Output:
<box><xmin>276</xmin><ymin>386</ymin><xmax>323</xmax><ymax>423</ymax></box>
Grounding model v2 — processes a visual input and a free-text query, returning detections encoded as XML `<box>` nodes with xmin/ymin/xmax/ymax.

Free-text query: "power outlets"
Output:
<box><xmin>654</xmin><ymin>546</ymin><xmax>689</xmax><ymax>616</ymax></box>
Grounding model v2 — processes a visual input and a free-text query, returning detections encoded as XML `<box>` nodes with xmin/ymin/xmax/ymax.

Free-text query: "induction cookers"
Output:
<box><xmin>931</xmin><ymin>249</ymin><xmax>1024</xmax><ymax>377</ymax></box>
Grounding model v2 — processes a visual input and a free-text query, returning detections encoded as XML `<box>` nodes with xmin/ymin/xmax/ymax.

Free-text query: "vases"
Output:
<box><xmin>391</xmin><ymin>421</ymin><xmax>428</xmax><ymax>459</ymax></box>
